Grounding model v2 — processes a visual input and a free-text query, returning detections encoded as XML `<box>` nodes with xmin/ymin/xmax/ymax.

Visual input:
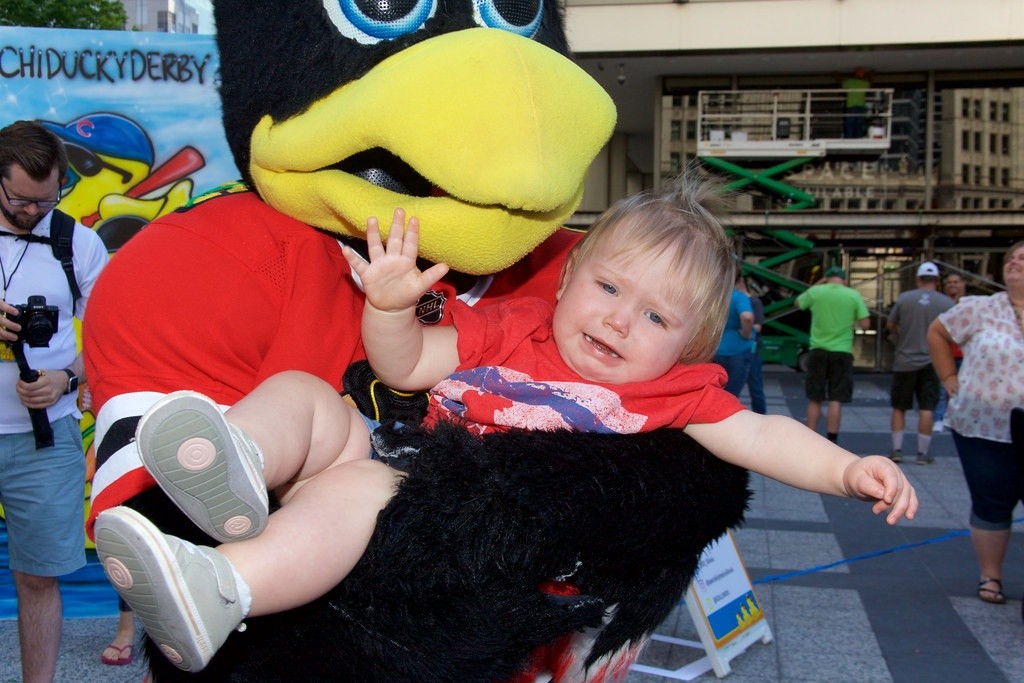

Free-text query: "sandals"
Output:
<box><xmin>977</xmin><ymin>579</ymin><xmax>1006</xmax><ymax>603</ymax></box>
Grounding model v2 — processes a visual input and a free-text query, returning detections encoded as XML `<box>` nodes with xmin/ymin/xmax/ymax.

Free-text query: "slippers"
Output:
<box><xmin>101</xmin><ymin>644</ymin><xmax>133</xmax><ymax>665</ymax></box>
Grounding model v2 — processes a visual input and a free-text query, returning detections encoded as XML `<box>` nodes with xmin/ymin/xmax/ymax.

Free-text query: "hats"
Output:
<box><xmin>917</xmin><ymin>262</ymin><xmax>939</xmax><ymax>277</ymax></box>
<box><xmin>824</xmin><ymin>267</ymin><xmax>846</xmax><ymax>279</ymax></box>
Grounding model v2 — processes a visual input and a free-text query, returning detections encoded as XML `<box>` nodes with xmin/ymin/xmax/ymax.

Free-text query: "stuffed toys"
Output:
<box><xmin>81</xmin><ymin>0</ymin><xmax>755</xmax><ymax>683</ymax></box>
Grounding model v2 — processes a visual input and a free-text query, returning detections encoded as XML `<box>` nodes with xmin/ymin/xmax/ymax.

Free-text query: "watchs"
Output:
<box><xmin>61</xmin><ymin>369</ymin><xmax>78</xmax><ymax>395</ymax></box>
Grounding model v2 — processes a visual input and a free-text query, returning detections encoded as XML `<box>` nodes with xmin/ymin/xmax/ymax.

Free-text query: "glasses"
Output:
<box><xmin>1</xmin><ymin>183</ymin><xmax>60</xmax><ymax>209</ymax></box>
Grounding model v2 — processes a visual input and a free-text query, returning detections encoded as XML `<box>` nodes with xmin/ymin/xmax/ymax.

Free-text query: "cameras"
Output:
<box><xmin>6</xmin><ymin>295</ymin><xmax>60</xmax><ymax>349</ymax></box>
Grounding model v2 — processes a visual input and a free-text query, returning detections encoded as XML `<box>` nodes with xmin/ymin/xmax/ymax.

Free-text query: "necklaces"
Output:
<box><xmin>1009</xmin><ymin>298</ymin><xmax>1024</xmax><ymax>332</ymax></box>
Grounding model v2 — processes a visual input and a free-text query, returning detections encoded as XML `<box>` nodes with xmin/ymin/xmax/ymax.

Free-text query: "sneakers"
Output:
<box><xmin>135</xmin><ymin>391</ymin><xmax>269</xmax><ymax>545</ymax></box>
<box><xmin>94</xmin><ymin>505</ymin><xmax>243</xmax><ymax>673</ymax></box>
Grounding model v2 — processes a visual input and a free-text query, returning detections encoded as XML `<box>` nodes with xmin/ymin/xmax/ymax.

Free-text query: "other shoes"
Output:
<box><xmin>916</xmin><ymin>453</ymin><xmax>937</xmax><ymax>465</ymax></box>
<box><xmin>932</xmin><ymin>420</ymin><xmax>944</xmax><ymax>433</ymax></box>
<box><xmin>888</xmin><ymin>449</ymin><xmax>904</xmax><ymax>461</ymax></box>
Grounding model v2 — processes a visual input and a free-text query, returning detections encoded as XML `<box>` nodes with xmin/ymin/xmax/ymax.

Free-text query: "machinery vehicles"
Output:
<box><xmin>699</xmin><ymin>85</ymin><xmax>895</xmax><ymax>371</ymax></box>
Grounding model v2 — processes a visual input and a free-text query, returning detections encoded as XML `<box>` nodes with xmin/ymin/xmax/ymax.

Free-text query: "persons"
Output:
<box><xmin>932</xmin><ymin>270</ymin><xmax>967</xmax><ymax>433</ymax></box>
<box><xmin>794</xmin><ymin>267</ymin><xmax>870</xmax><ymax>445</ymax></box>
<box><xmin>92</xmin><ymin>156</ymin><xmax>919</xmax><ymax>672</ymax></box>
<box><xmin>100</xmin><ymin>595</ymin><xmax>136</xmax><ymax>665</ymax></box>
<box><xmin>927</xmin><ymin>241</ymin><xmax>1024</xmax><ymax>604</ymax></box>
<box><xmin>0</xmin><ymin>119</ymin><xmax>110</xmax><ymax>683</ymax></box>
<box><xmin>713</xmin><ymin>266</ymin><xmax>767</xmax><ymax>415</ymax></box>
<box><xmin>886</xmin><ymin>262</ymin><xmax>955</xmax><ymax>465</ymax></box>
